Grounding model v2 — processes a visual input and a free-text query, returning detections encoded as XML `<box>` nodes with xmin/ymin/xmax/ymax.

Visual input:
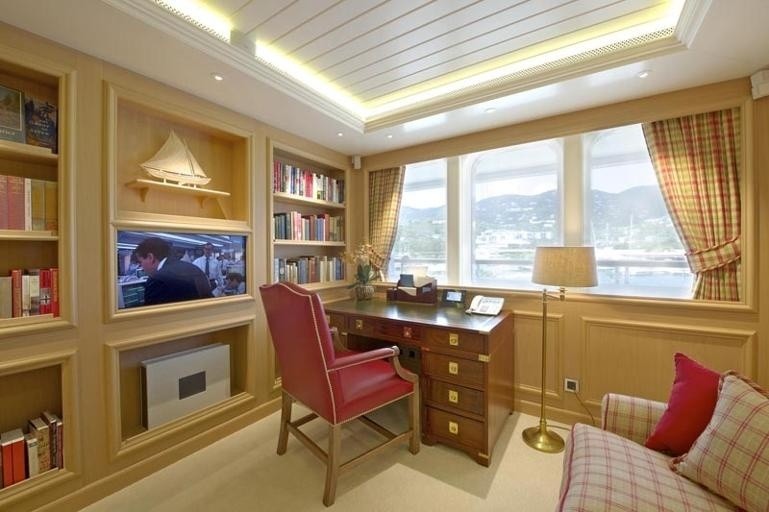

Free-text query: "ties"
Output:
<box><xmin>205</xmin><ymin>257</ymin><xmax>209</xmax><ymax>277</ymax></box>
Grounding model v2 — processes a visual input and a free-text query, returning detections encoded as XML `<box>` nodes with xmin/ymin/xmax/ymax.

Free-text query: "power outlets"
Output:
<box><xmin>564</xmin><ymin>377</ymin><xmax>579</xmax><ymax>394</ymax></box>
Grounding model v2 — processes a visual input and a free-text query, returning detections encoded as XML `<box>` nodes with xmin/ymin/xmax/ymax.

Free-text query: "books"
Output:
<box><xmin>0</xmin><ymin>84</ymin><xmax>63</xmax><ymax>487</ymax></box>
<box><xmin>274</xmin><ymin>159</ymin><xmax>345</xmax><ymax>284</ymax></box>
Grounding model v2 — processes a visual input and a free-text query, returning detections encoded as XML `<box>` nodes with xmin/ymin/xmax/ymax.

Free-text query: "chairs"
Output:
<box><xmin>259</xmin><ymin>282</ymin><xmax>420</xmax><ymax>507</ymax></box>
<box><xmin>558</xmin><ymin>351</ymin><xmax>768</xmax><ymax>511</ymax></box>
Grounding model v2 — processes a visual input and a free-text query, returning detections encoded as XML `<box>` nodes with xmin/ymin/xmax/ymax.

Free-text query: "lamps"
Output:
<box><xmin>521</xmin><ymin>246</ymin><xmax>599</xmax><ymax>454</ymax></box>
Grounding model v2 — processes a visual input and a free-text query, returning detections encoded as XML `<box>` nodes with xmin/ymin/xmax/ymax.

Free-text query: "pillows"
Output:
<box><xmin>671</xmin><ymin>370</ymin><xmax>768</xmax><ymax>511</ymax></box>
<box><xmin>646</xmin><ymin>353</ymin><xmax>724</xmax><ymax>455</ymax></box>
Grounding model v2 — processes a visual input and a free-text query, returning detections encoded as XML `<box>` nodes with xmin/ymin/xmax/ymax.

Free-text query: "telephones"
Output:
<box><xmin>465</xmin><ymin>295</ymin><xmax>505</xmax><ymax>316</ymax></box>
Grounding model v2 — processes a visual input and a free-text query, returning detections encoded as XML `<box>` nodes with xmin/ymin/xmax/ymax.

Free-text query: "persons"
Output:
<box><xmin>129</xmin><ymin>237</ymin><xmax>246</xmax><ymax>306</ymax></box>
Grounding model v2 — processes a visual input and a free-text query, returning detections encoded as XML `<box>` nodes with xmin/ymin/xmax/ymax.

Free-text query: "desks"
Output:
<box><xmin>321</xmin><ymin>293</ymin><xmax>518</xmax><ymax>467</ymax></box>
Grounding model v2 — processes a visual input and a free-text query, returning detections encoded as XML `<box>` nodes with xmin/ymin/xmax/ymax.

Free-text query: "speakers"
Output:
<box><xmin>750</xmin><ymin>69</ymin><xmax>769</xmax><ymax>100</ymax></box>
<box><xmin>353</xmin><ymin>155</ymin><xmax>361</xmax><ymax>170</ymax></box>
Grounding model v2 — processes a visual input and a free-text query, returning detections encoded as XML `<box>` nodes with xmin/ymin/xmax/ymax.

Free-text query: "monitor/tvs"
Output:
<box><xmin>116</xmin><ymin>227</ymin><xmax>247</xmax><ymax>313</ymax></box>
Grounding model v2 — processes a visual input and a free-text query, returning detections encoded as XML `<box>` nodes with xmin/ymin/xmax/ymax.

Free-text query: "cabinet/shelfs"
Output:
<box><xmin>268</xmin><ymin>143</ymin><xmax>351</xmax><ymax>393</ymax></box>
<box><xmin>1</xmin><ymin>25</ymin><xmax>80</xmax><ymax>510</ymax></box>
